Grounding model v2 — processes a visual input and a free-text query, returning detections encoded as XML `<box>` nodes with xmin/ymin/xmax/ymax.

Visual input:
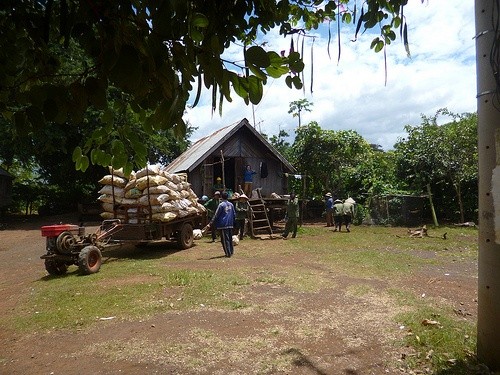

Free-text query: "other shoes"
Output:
<box><xmin>334</xmin><ymin>227</ymin><xmax>350</xmax><ymax>232</ymax></box>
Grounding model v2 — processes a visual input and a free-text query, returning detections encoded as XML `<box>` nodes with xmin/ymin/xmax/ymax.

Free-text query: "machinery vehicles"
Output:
<box><xmin>40</xmin><ymin>215</ymin><xmax>203</xmax><ymax>277</ymax></box>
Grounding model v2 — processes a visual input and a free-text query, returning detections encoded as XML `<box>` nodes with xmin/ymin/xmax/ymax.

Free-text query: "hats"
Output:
<box><xmin>333</xmin><ymin>197</ymin><xmax>356</xmax><ymax>204</ymax></box>
<box><xmin>239</xmin><ymin>194</ymin><xmax>249</xmax><ymax>200</ymax></box>
<box><xmin>325</xmin><ymin>193</ymin><xmax>332</xmax><ymax>197</ymax></box>
<box><xmin>213</xmin><ymin>191</ymin><xmax>220</xmax><ymax>195</ymax></box>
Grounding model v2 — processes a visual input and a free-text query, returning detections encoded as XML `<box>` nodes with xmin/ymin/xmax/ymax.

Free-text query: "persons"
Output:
<box><xmin>324</xmin><ymin>192</ymin><xmax>357</xmax><ymax>232</ymax></box>
<box><xmin>199</xmin><ymin>164</ymin><xmax>300</xmax><ymax>258</ymax></box>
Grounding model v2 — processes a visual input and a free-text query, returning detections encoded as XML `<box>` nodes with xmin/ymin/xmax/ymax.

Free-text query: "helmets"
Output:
<box><xmin>216</xmin><ymin>177</ymin><xmax>222</xmax><ymax>180</ymax></box>
